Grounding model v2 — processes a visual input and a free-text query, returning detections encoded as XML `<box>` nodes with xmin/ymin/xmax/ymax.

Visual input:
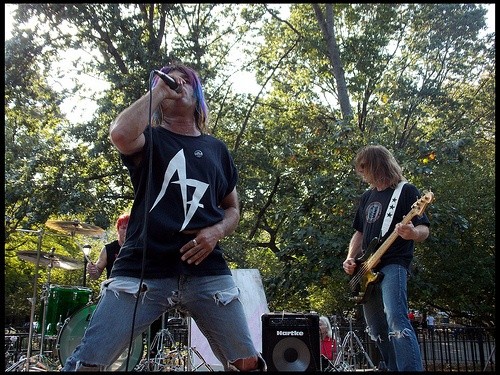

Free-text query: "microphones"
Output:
<box><xmin>154</xmin><ymin>69</ymin><xmax>181</xmax><ymax>92</ymax></box>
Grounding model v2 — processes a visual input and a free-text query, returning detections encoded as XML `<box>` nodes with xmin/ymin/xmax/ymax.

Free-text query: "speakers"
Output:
<box><xmin>262</xmin><ymin>312</ymin><xmax>320</xmax><ymax>371</ymax></box>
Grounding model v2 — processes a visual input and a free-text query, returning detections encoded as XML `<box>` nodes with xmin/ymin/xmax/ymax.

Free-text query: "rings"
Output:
<box><xmin>192</xmin><ymin>238</ymin><xmax>197</xmax><ymax>246</ymax></box>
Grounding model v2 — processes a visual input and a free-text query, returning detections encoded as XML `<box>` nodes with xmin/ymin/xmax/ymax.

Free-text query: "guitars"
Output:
<box><xmin>345</xmin><ymin>189</ymin><xmax>436</xmax><ymax>306</ymax></box>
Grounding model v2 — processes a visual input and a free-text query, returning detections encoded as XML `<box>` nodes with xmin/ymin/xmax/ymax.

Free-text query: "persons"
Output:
<box><xmin>426</xmin><ymin>312</ymin><xmax>433</xmax><ymax>331</ymax></box>
<box><xmin>59</xmin><ymin>61</ymin><xmax>273</xmax><ymax>375</ymax></box>
<box><xmin>343</xmin><ymin>143</ymin><xmax>433</xmax><ymax>374</ymax></box>
<box><xmin>406</xmin><ymin>309</ymin><xmax>416</xmax><ymax>320</ymax></box>
<box><xmin>316</xmin><ymin>315</ymin><xmax>340</xmax><ymax>373</ymax></box>
<box><xmin>85</xmin><ymin>213</ymin><xmax>168</xmax><ymax>374</ymax></box>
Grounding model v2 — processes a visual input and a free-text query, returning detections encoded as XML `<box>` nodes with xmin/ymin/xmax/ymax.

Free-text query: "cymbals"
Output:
<box><xmin>45</xmin><ymin>217</ymin><xmax>104</xmax><ymax>237</ymax></box>
<box><xmin>15</xmin><ymin>245</ymin><xmax>85</xmax><ymax>273</ymax></box>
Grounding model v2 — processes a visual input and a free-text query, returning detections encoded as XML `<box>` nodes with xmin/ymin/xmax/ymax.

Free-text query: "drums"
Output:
<box><xmin>56</xmin><ymin>302</ymin><xmax>148</xmax><ymax>372</ymax></box>
<box><xmin>35</xmin><ymin>283</ymin><xmax>97</xmax><ymax>338</ymax></box>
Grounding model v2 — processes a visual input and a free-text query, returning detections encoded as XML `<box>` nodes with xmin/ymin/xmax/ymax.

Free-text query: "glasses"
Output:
<box><xmin>359</xmin><ymin>161</ymin><xmax>367</xmax><ymax>169</ymax></box>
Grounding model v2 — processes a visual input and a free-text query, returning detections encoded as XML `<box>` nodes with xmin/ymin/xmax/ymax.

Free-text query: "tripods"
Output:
<box><xmin>136</xmin><ymin>311</ymin><xmax>185</xmax><ymax>373</ymax></box>
<box><xmin>333</xmin><ymin>318</ymin><xmax>377</xmax><ymax>372</ymax></box>
<box><xmin>5</xmin><ymin>234</ymin><xmax>63</xmax><ymax>373</ymax></box>
